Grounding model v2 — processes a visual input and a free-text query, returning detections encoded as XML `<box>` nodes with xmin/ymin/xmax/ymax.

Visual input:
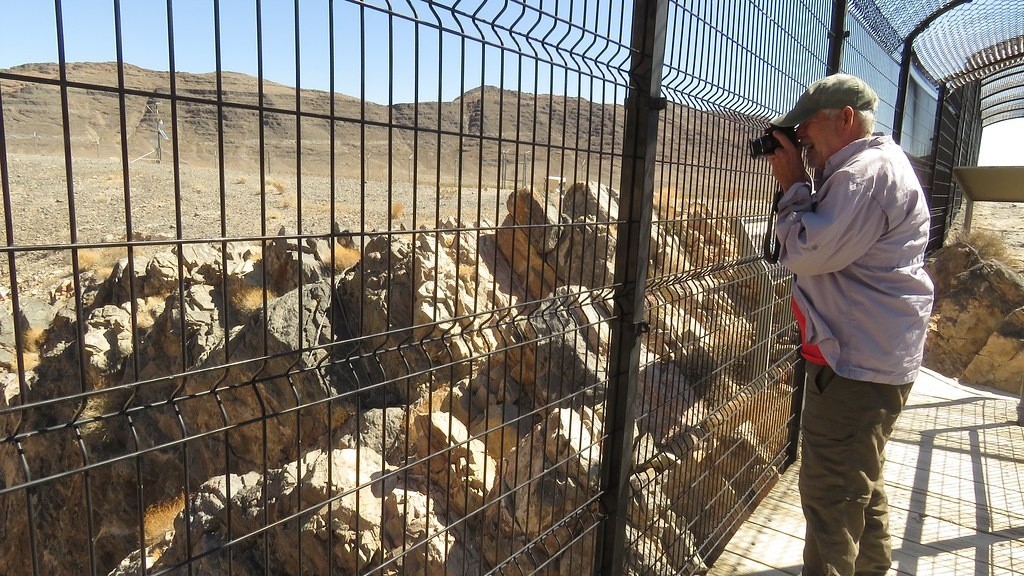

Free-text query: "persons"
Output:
<box><xmin>776</xmin><ymin>74</ymin><xmax>937</xmax><ymax>576</ymax></box>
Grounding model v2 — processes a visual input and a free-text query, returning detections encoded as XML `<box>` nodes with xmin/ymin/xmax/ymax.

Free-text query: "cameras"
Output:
<box><xmin>749</xmin><ymin>124</ymin><xmax>798</xmax><ymax>160</ymax></box>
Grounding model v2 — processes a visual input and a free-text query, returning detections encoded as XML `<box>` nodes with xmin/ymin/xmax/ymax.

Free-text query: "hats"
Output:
<box><xmin>769</xmin><ymin>73</ymin><xmax>879</xmax><ymax>128</ymax></box>
